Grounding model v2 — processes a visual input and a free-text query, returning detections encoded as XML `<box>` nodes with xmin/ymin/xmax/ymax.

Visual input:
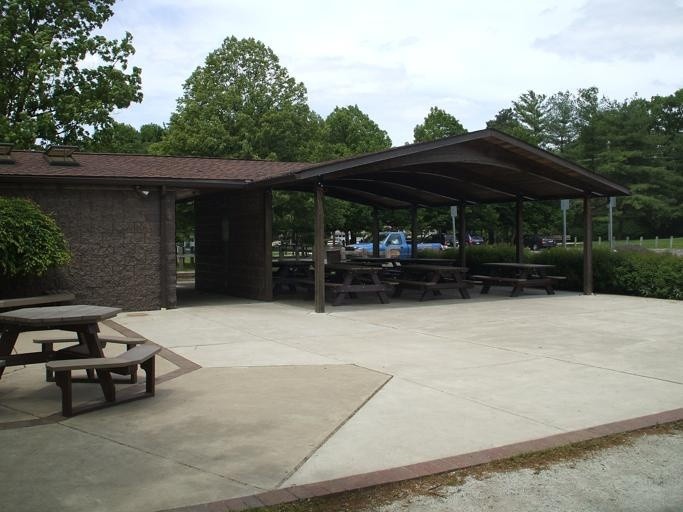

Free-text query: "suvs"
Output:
<box><xmin>421</xmin><ymin>233</ymin><xmax>460</xmax><ymax>250</ymax></box>
<box><xmin>454</xmin><ymin>231</ymin><xmax>483</xmax><ymax>247</ymax></box>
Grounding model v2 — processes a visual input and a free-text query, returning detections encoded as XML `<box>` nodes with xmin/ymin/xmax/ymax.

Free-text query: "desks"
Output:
<box><xmin>0</xmin><ymin>304</ymin><xmax>123</xmax><ymax>417</ymax></box>
<box><xmin>271</xmin><ymin>256</ymin><xmax>568</xmax><ymax>306</ymax></box>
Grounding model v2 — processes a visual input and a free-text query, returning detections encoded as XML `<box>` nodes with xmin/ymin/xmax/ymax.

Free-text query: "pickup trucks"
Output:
<box><xmin>345</xmin><ymin>229</ymin><xmax>443</xmax><ymax>261</ymax></box>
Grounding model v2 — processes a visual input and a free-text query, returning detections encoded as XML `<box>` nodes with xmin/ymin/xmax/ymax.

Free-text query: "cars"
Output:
<box><xmin>521</xmin><ymin>233</ymin><xmax>555</xmax><ymax>251</ymax></box>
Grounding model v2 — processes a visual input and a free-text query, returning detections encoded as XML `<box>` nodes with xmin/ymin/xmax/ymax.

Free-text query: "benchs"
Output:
<box><xmin>32</xmin><ymin>334</ymin><xmax>145</xmax><ymax>385</ymax></box>
<box><xmin>46</xmin><ymin>342</ymin><xmax>163</xmax><ymax>417</ymax></box>
